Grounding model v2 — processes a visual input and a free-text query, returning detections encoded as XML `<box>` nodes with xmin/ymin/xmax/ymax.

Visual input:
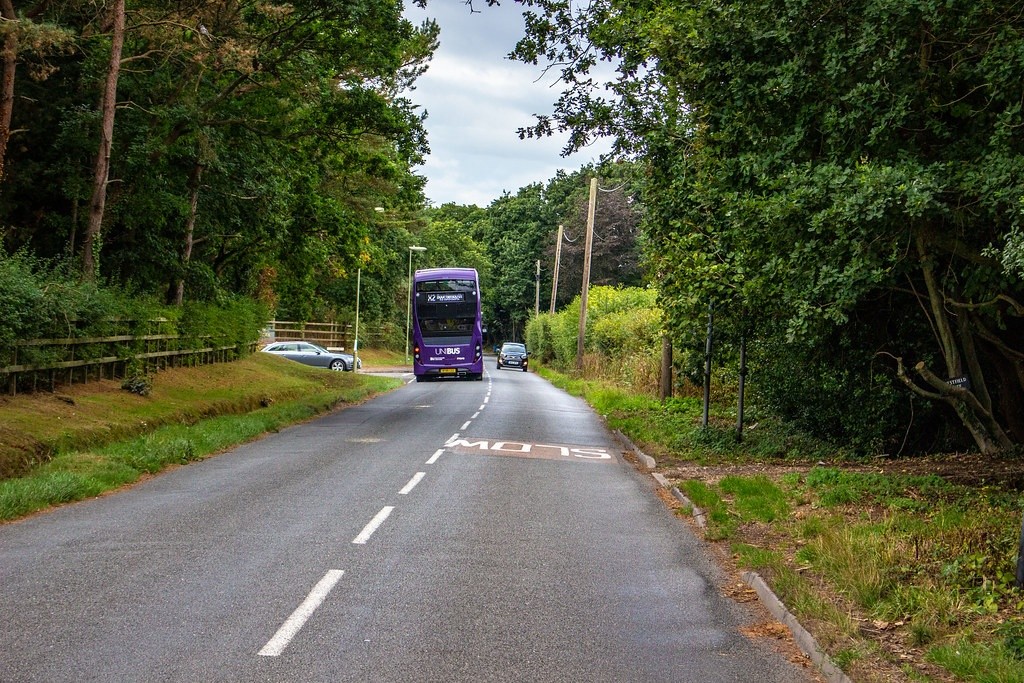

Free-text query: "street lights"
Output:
<box><xmin>405</xmin><ymin>244</ymin><xmax>428</xmax><ymax>360</ymax></box>
<box><xmin>352</xmin><ymin>207</ymin><xmax>385</xmax><ymax>373</ymax></box>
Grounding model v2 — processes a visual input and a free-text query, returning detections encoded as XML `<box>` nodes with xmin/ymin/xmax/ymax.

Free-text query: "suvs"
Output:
<box><xmin>496</xmin><ymin>342</ymin><xmax>532</xmax><ymax>372</ymax></box>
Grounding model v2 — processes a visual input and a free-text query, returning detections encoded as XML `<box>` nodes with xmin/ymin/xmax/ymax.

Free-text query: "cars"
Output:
<box><xmin>259</xmin><ymin>340</ymin><xmax>362</xmax><ymax>372</ymax></box>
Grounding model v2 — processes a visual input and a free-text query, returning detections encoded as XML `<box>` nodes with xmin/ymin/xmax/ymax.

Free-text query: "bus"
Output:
<box><xmin>410</xmin><ymin>267</ymin><xmax>484</xmax><ymax>383</ymax></box>
